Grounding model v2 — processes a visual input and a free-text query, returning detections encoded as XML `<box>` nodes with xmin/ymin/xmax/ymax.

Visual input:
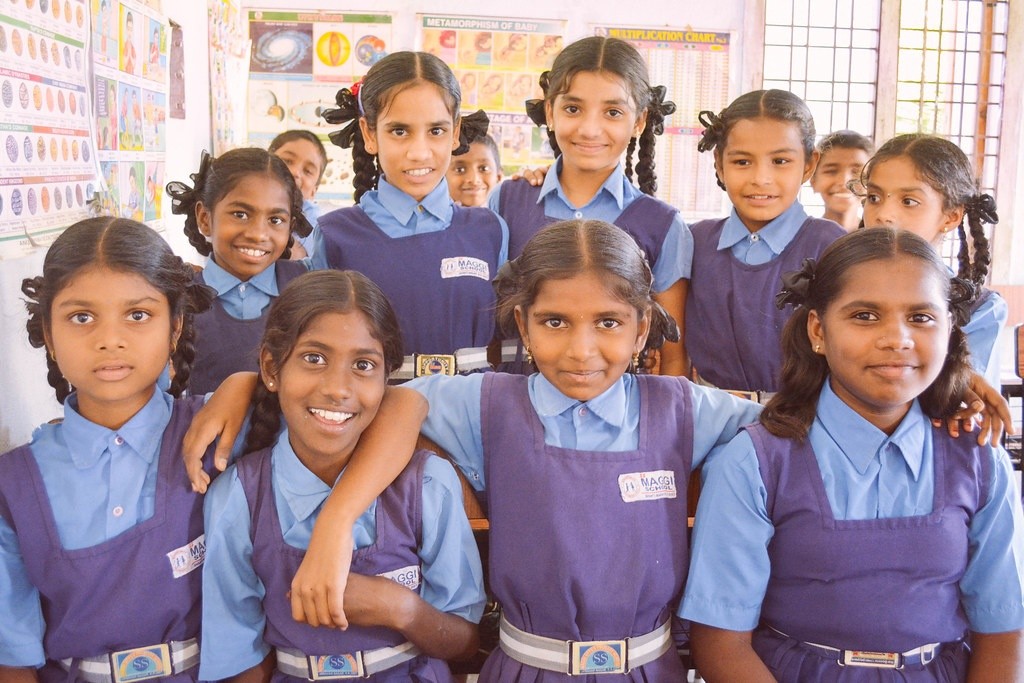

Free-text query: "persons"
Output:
<box><xmin>850</xmin><ymin>131</ymin><xmax>1007</xmax><ymax>438</ymax></box>
<box><xmin>166</xmin><ymin>147</ymin><xmax>314</xmax><ymax>394</ymax></box>
<box><xmin>486</xmin><ymin>33</ymin><xmax>695</xmax><ymax>406</ymax></box>
<box><xmin>197</xmin><ymin>268</ymin><xmax>487</xmax><ymax>682</ymax></box>
<box><xmin>0</xmin><ymin>216</ymin><xmax>431</xmax><ymax>683</ymax></box>
<box><xmin>313</xmin><ymin>49</ymin><xmax>550</xmax><ymax>380</ymax></box>
<box><xmin>677</xmin><ymin>229</ymin><xmax>1024</xmax><ymax>683</ymax></box>
<box><xmin>183</xmin><ymin>217</ymin><xmax>1017</xmax><ymax>682</ymax></box>
<box><xmin>683</xmin><ymin>90</ymin><xmax>850</xmax><ymax>409</ymax></box>
<box><xmin>445</xmin><ymin>131</ymin><xmax>504</xmax><ymax>211</ymax></box>
<box><xmin>266</xmin><ymin>130</ymin><xmax>329</xmax><ymax>262</ymax></box>
<box><xmin>807</xmin><ymin>131</ymin><xmax>874</xmax><ymax>235</ymax></box>
